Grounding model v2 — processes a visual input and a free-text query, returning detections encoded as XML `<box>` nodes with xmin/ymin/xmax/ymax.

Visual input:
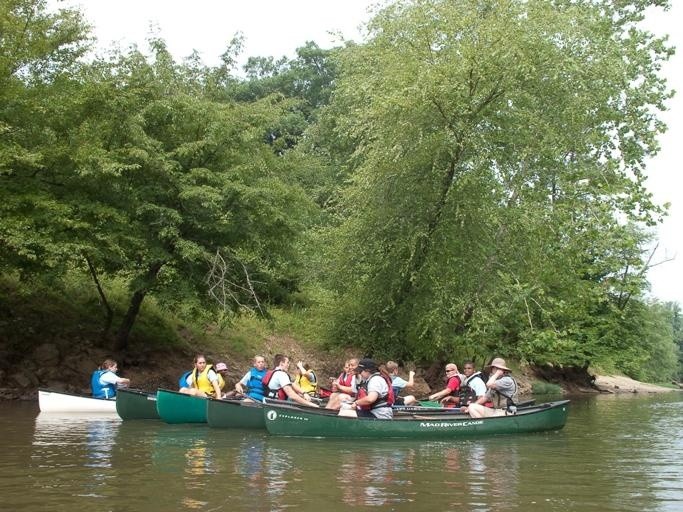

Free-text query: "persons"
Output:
<box><xmin>90</xmin><ymin>359</ymin><xmax>131</xmax><ymax>400</ymax></box>
<box><xmin>464</xmin><ymin>357</ymin><xmax>518</xmax><ymax>418</ymax></box>
<box><xmin>179</xmin><ymin>351</ymin><xmax>416</xmax><ymax>420</ymax></box>
<box><xmin>440</xmin><ymin>360</ymin><xmax>488</xmax><ymax>409</ymax></box>
<box><xmin>417</xmin><ymin>363</ymin><xmax>466</xmax><ymax>408</ymax></box>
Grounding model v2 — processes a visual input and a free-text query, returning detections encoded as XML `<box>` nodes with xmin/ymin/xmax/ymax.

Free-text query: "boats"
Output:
<box><xmin>37</xmin><ymin>389</ymin><xmax>118</xmax><ymax>417</ymax></box>
<box><xmin>260</xmin><ymin>398</ymin><xmax>570</xmax><ymax>441</ymax></box>
<box><xmin>206</xmin><ymin>398</ymin><xmax>538</xmax><ymax>432</ymax></box>
<box><xmin>155</xmin><ymin>387</ymin><xmax>212</xmax><ymax>424</ymax></box>
<box><xmin>114</xmin><ymin>388</ymin><xmax>159</xmax><ymax>423</ymax></box>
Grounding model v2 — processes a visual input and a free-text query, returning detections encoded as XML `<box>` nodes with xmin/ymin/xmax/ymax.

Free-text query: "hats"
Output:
<box><xmin>352</xmin><ymin>359</ymin><xmax>376</xmax><ymax>371</ymax></box>
<box><xmin>483</xmin><ymin>357</ymin><xmax>512</xmax><ymax>375</ymax></box>
<box><xmin>385</xmin><ymin>361</ymin><xmax>398</xmax><ymax>372</ymax></box>
<box><xmin>215</xmin><ymin>363</ymin><xmax>228</xmax><ymax>373</ymax></box>
